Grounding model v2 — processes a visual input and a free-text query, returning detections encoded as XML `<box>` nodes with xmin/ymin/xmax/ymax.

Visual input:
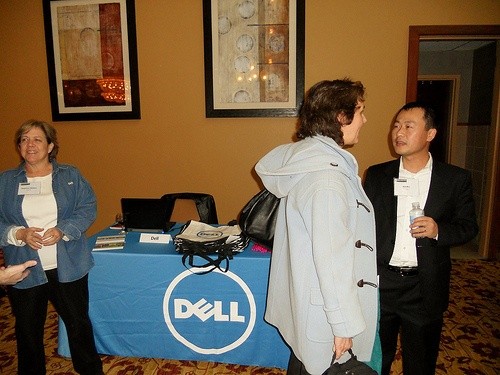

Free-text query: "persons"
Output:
<box><xmin>255</xmin><ymin>77</ymin><xmax>381</xmax><ymax>375</ymax></box>
<box><xmin>361</xmin><ymin>102</ymin><xmax>479</xmax><ymax>375</ymax></box>
<box><xmin>0</xmin><ymin>119</ymin><xmax>104</xmax><ymax>375</ymax></box>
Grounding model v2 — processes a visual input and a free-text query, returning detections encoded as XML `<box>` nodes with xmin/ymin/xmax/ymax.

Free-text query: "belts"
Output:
<box><xmin>387</xmin><ymin>265</ymin><xmax>418</xmax><ymax>277</ymax></box>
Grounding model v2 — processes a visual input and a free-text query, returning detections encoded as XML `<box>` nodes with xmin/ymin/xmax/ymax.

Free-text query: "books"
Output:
<box><xmin>92</xmin><ymin>235</ymin><xmax>126</xmax><ymax>251</ymax></box>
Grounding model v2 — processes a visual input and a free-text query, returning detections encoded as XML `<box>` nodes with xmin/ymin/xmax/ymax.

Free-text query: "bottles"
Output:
<box><xmin>410</xmin><ymin>202</ymin><xmax>425</xmax><ymax>238</ymax></box>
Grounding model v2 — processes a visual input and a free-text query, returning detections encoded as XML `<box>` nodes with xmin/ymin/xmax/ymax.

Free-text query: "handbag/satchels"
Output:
<box><xmin>239</xmin><ymin>189</ymin><xmax>281</xmax><ymax>246</ymax></box>
<box><xmin>322</xmin><ymin>349</ymin><xmax>378</xmax><ymax>375</ymax></box>
<box><xmin>173</xmin><ymin>219</ymin><xmax>252</xmax><ymax>275</ymax></box>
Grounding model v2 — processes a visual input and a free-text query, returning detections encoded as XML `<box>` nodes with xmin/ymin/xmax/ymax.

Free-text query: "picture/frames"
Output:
<box><xmin>42</xmin><ymin>0</ymin><xmax>142</xmax><ymax>122</ymax></box>
<box><xmin>203</xmin><ymin>0</ymin><xmax>306</xmax><ymax>117</ymax></box>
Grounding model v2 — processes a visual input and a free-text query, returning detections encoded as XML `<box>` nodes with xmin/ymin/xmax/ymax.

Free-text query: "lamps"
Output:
<box><xmin>96</xmin><ymin>78</ymin><xmax>131</xmax><ymax>104</ymax></box>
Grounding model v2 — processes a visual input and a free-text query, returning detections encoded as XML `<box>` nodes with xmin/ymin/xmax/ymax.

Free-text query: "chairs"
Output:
<box><xmin>163</xmin><ymin>193</ymin><xmax>219</xmax><ymax>225</ymax></box>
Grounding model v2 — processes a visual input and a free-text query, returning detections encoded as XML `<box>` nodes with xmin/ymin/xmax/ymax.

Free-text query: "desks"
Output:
<box><xmin>52</xmin><ymin>221</ymin><xmax>292</xmax><ymax>375</ymax></box>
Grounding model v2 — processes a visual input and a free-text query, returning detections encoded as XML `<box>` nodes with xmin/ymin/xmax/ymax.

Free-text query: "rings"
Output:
<box><xmin>425</xmin><ymin>228</ymin><xmax>426</xmax><ymax>232</ymax></box>
<box><xmin>341</xmin><ymin>351</ymin><xmax>344</xmax><ymax>354</ymax></box>
